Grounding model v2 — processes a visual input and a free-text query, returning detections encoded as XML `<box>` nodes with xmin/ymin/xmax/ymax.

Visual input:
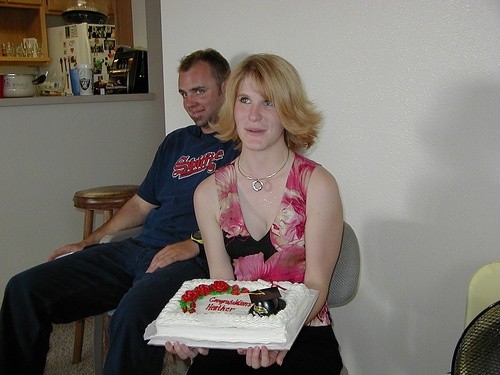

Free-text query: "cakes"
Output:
<box><xmin>156</xmin><ymin>278</ymin><xmax>310</xmax><ymax>343</ymax></box>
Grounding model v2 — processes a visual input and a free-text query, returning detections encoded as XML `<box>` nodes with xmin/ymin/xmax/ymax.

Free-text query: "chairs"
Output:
<box><xmin>93</xmin><ymin>226</ymin><xmax>191</xmax><ymax>375</ymax></box>
<box><xmin>325</xmin><ymin>221</ymin><xmax>360</xmax><ymax>375</ymax></box>
<box><xmin>450</xmin><ymin>261</ymin><xmax>500</xmax><ymax>375</ymax></box>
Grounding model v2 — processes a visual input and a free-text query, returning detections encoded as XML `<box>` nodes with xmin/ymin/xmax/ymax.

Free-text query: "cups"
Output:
<box><xmin>77</xmin><ymin>64</ymin><xmax>94</xmax><ymax>96</ymax></box>
<box><xmin>2</xmin><ymin>41</ymin><xmax>43</xmax><ymax>58</ymax></box>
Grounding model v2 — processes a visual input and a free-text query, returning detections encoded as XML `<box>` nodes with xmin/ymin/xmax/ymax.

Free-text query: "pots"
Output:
<box><xmin>4</xmin><ymin>74</ymin><xmax>46</xmax><ymax>98</ymax></box>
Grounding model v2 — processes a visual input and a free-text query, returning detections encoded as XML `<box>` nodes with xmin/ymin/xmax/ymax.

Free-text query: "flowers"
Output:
<box><xmin>180</xmin><ymin>280</ymin><xmax>249</xmax><ymax>315</ymax></box>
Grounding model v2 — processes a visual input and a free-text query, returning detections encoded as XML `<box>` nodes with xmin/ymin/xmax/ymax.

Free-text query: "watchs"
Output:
<box><xmin>191</xmin><ymin>230</ymin><xmax>204</xmax><ymax>255</ymax></box>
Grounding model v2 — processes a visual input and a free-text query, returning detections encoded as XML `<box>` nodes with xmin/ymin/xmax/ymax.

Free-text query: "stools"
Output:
<box><xmin>71</xmin><ymin>185</ymin><xmax>141</xmax><ymax>364</ymax></box>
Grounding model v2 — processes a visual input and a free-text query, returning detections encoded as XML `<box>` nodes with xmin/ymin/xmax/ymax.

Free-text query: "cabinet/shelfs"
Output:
<box><xmin>0</xmin><ymin>0</ymin><xmax>134</xmax><ymax>66</ymax></box>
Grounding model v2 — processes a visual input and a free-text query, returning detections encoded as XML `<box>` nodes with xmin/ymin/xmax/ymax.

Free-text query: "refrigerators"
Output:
<box><xmin>39</xmin><ymin>23</ymin><xmax>116</xmax><ymax>91</ymax></box>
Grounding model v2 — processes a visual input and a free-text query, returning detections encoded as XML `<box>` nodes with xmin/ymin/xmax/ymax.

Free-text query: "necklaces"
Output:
<box><xmin>238</xmin><ymin>149</ymin><xmax>289</xmax><ymax>192</ymax></box>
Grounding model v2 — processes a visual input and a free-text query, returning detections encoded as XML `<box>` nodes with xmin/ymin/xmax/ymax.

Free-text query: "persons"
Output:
<box><xmin>164</xmin><ymin>52</ymin><xmax>344</xmax><ymax>375</ymax></box>
<box><xmin>0</xmin><ymin>47</ymin><xmax>242</xmax><ymax>375</ymax></box>
